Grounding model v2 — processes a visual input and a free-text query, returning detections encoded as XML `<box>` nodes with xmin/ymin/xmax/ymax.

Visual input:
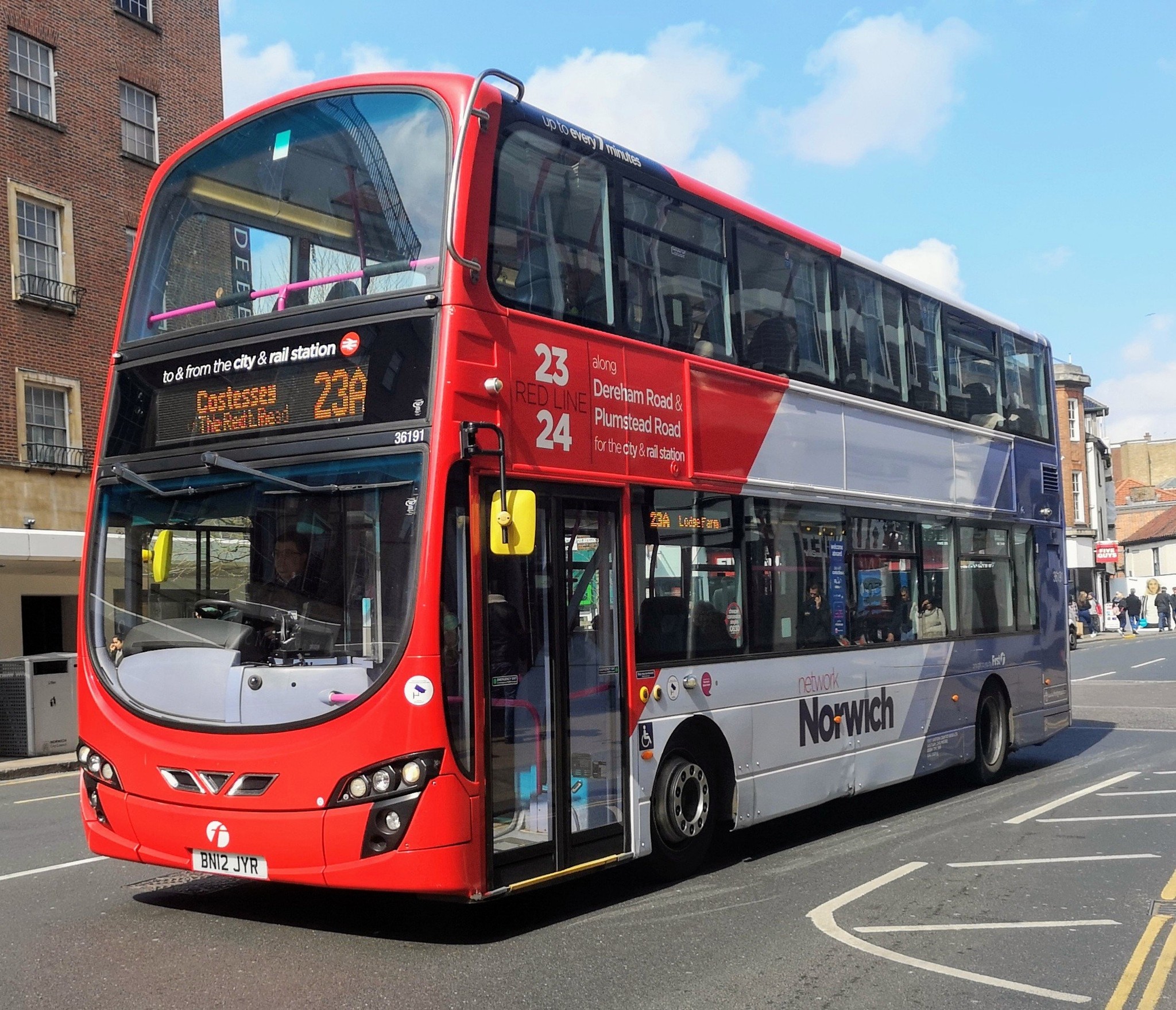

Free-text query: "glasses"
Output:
<box><xmin>272</xmin><ymin>549</ymin><xmax>303</xmax><ymax>558</ymax></box>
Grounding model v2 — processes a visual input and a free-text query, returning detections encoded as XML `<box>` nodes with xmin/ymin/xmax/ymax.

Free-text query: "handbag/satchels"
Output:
<box><xmin>1076</xmin><ymin>621</ymin><xmax>1084</xmax><ymax>636</ymax></box>
<box><xmin>1093</xmin><ymin>598</ymin><xmax>1102</xmax><ymax>616</ymax></box>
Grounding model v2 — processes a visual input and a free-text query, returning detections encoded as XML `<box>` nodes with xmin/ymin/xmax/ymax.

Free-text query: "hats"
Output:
<box><xmin>1116</xmin><ymin>591</ymin><xmax>1119</xmax><ymax>595</ymax></box>
<box><xmin>1088</xmin><ymin>592</ymin><xmax>1094</xmax><ymax>597</ymax></box>
<box><xmin>1130</xmin><ymin>588</ymin><xmax>1135</xmax><ymax>592</ymax></box>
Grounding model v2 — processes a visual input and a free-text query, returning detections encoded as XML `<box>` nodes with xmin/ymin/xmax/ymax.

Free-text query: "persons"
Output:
<box><xmin>1069</xmin><ymin>587</ymin><xmax>1176</xmax><ymax>638</ymax></box>
<box><xmin>487</xmin><ymin>580</ymin><xmax>527</xmax><ymax>744</ymax></box>
<box><xmin>918</xmin><ymin>593</ymin><xmax>947</xmax><ymax>639</ymax></box>
<box><xmin>800</xmin><ymin>580</ymin><xmax>832</xmax><ymax>648</ymax></box>
<box><xmin>107</xmin><ymin>633</ymin><xmax>124</xmax><ymax>667</ymax></box>
<box><xmin>193</xmin><ymin>528</ymin><xmax>320</xmax><ymax>660</ymax></box>
<box><xmin>886</xmin><ymin>584</ymin><xmax>918</xmax><ymax>642</ymax></box>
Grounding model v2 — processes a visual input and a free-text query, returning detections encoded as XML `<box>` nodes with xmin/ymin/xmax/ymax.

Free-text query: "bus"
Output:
<box><xmin>75</xmin><ymin>68</ymin><xmax>1074</xmax><ymax>906</ymax></box>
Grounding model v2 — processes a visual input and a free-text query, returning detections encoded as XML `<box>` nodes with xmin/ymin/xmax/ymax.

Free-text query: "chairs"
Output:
<box><xmin>486</xmin><ymin>590</ymin><xmax>928</xmax><ymax>673</ymax></box>
<box><xmin>514</xmin><ymin>249</ymin><xmax>1045</xmax><ymax>436</ymax></box>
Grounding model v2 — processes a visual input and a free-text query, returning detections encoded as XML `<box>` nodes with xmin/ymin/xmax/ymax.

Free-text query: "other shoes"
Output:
<box><xmin>1132</xmin><ymin>629</ymin><xmax>1139</xmax><ymax>634</ymax></box>
<box><xmin>1159</xmin><ymin>629</ymin><xmax>1162</xmax><ymax>632</ymax></box>
<box><xmin>1091</xmin><ymin>633</ymin><xmax>1096</xmax><ymax>638</ymax></box>
<box><xmin>1117</xmin><ymin>627</ymin><xmax>1124</xmax><ymax>636</ymax></box>
<box><xmin>1169</xmin><ymin>629</ymin><xmax>1172</xmax><ymax>631</ymax></box>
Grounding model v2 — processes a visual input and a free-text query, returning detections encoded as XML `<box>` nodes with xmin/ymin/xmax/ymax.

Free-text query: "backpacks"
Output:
<box><xmin>1113</xmin><ymin>603</ymin><xmax>1121</xmax><ymax>615</ymax></box>
